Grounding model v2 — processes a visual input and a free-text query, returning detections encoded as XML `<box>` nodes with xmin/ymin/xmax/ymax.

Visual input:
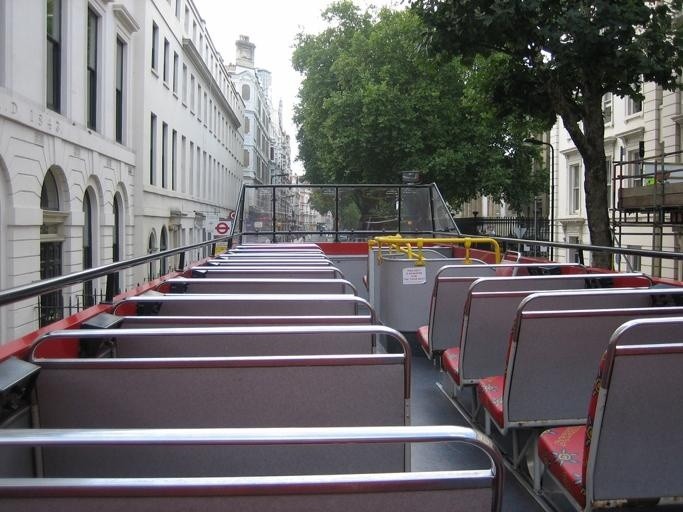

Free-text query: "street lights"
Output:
<box><xmin>523</xmin><ymin>138</ymin><xmax>554</xmax><ymax>261</ymax></box>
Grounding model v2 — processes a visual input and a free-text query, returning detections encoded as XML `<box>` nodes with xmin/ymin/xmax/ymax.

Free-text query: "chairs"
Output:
<box><xmin>1</xmin><ymin>244</ymin><xmax>506</xmax><ymax>511</ymax></box>
<box><xmin>418</xmin><ymin>264</ymin><xmax>683</xmax><ymax>511</ymax></box>
